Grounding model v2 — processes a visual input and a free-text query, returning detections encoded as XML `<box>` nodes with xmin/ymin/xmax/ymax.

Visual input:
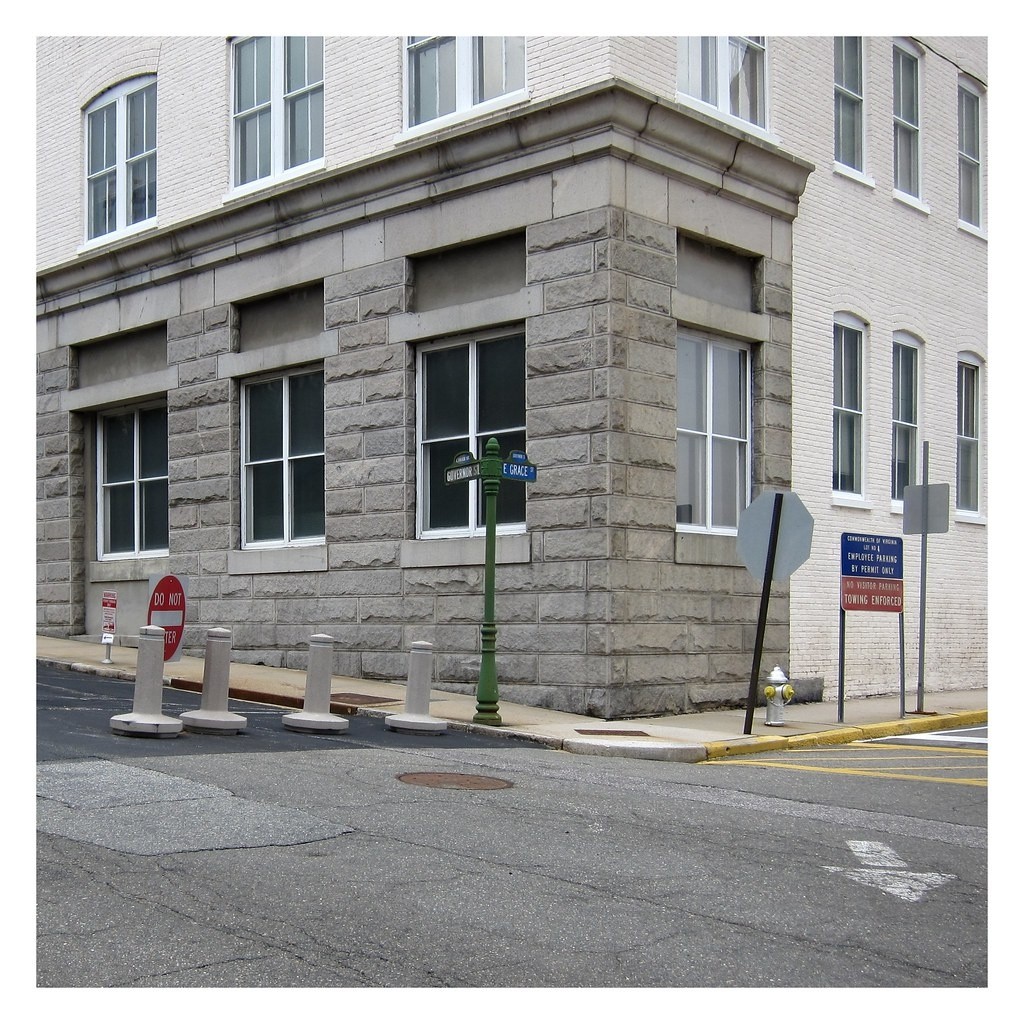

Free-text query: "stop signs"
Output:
<box><xmin>148</xmin><ymin>573</ymin><xmax>188</xmax><ymax>663</ymax></box>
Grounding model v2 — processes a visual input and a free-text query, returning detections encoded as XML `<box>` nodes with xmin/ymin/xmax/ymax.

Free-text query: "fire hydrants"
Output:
<box><xmin>764</xmin><ymin>664</ymin><xmax>795</xmax><ymax>727</ymax></box>
